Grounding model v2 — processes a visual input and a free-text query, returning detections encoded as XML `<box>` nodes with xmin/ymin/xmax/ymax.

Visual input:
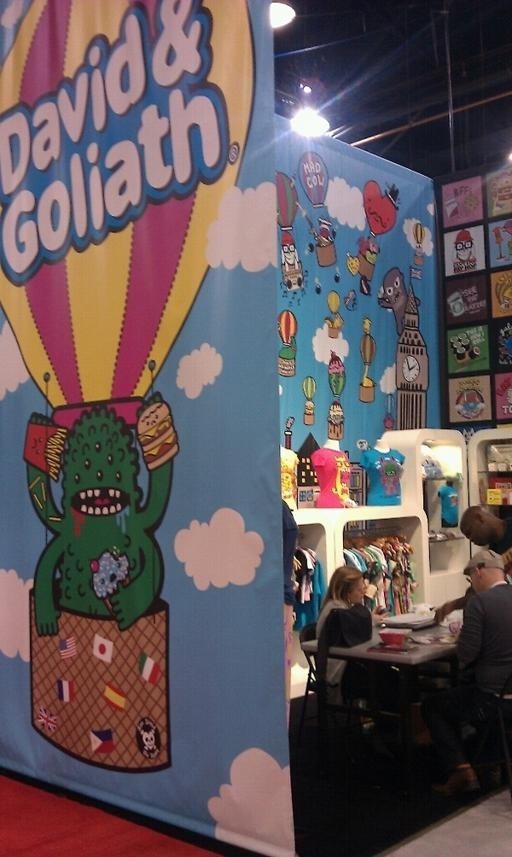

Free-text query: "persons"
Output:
<box><xmin>437</xmin><ymin>480</ymin><xmax>460</xmax><ymax>527</ymax></box>
<box><xmin>280</xmin><ymin>446</ymin><xmax>300</xmax><ymax>510</ymax></box>
<box><xmin>419</xmin><ymin>550</ymin><xmax>512</xmax><ymax>789</ymax></box>
<box><xmin>360</xmin><ymin>440</ymin><xmax>405</xmax><ymax>506</ymax></box>
<box><xmin>316</xmin><ymin>568</ymin><xmax>426</xmax><ymax>761</ymax></box>
<box><xmin>429</xmin><ymin>505</ymin><xmax>512</xmax><ymax>624</ymax></box>
<box><xmin>310</xmin><ymin>439</ymin><xmax>352</xmax><ymax>508</ymax></box>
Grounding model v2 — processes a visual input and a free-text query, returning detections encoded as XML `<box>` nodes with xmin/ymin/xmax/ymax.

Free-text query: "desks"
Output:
<box><xmin>301</xmin><ymin>625</ymin><xmax>456</xmax><ymax>800</ymax></box>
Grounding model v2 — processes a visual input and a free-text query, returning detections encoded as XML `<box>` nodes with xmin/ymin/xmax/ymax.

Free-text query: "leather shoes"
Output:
<box><xmin>434</xmin><ymin>769</ymin><xmax>480</xmax><ymax>799</ymax></box>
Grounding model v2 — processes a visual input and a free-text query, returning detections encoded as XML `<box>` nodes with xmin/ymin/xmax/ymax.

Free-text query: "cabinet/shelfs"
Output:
<box><xmin>468</xmin><ymin>428</ymin><xmax>512</xmax><ymax>550</ymax></box>
<box><xmin>381</xmin><ymin>429</ymin><xmax>469</xmax><ymax>607</ymax></box>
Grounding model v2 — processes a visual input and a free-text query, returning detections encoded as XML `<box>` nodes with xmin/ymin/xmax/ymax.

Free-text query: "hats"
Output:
<box><xmin>464</xmin><ymin>549</ymin><xmax>504</xmax><ymax>575</ymax></box>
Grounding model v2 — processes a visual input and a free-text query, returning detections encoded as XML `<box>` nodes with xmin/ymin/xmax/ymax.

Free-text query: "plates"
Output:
<box><xmin>378</xmin><ymin>613</ymin><xmax>438</xmax><ymax>630</ymax></box>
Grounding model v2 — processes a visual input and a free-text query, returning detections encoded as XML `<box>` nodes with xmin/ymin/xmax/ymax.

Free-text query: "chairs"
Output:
<box><xmin>298</xmin><ymin>623</ymin><xmax>350</xmax><ymax>748</ymax></box>
<box><xmin>447</xmin><ymin>674</ymin><xmax>511</xmax><ymax>795</ymax></box>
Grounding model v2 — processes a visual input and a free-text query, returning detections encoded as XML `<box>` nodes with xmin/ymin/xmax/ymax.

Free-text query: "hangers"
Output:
<box><xmin>368</xmin><ymin>528</ymin><xmax>414</xmax><ymax>555</ymax></box>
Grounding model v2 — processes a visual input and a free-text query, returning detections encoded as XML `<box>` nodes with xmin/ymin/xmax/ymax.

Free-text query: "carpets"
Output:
<box><xmin>289</xmin><ymin>694</ymin><xmax>510</xmax><ymax>857</ymax></box>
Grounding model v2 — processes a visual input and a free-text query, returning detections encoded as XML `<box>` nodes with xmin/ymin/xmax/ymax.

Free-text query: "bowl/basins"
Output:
<box><xmin>379</xmin><ymin>627</ymin><xmax>412</xmax><ymax>644</ymax></box>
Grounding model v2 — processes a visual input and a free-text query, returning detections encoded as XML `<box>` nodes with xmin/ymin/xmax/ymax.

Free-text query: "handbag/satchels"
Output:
<box><xmin>327</xmin><ymin>602</ymin><xmax>373</xmax><ymax>648</ymax></box>
